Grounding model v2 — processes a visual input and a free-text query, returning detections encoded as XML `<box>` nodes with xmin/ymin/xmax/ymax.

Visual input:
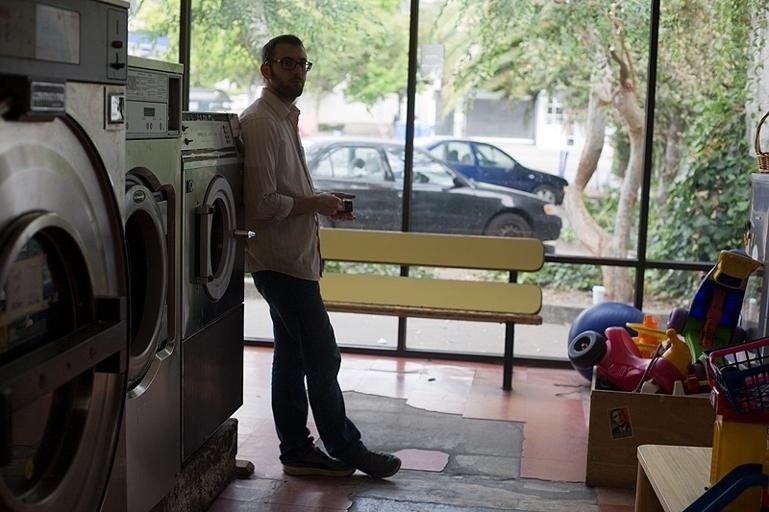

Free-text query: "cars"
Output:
<box><xmin>388</xmin><ymin>136</ymin><xmax>567</xmax><ymax>206</ymax></box>
<box><xmin>298</xmin><ymin>136</ymin><xmax>566</xmax><ymax>257</ymax></box>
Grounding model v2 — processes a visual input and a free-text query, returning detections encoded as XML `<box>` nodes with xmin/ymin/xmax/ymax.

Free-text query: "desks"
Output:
<box><xmin>628</xmin><ymin>444</ymin><xmax>713</xmax><ymax>512</ymax></box>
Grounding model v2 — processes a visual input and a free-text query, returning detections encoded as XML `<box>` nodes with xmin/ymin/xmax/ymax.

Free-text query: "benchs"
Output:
<box><xmin>319</xmin><ymin>224</ymin><xmax>545</xmax><ymax>395</ymax></box>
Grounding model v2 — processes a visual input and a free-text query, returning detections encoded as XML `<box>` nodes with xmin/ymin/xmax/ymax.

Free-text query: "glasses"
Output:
<box><xmin>267</xmin><ymin>57</ymin><xmax>313</xmax><ymax>74</ymax></box>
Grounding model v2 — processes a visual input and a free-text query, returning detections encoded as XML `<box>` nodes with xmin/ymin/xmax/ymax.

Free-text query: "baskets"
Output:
<box><xmin>708</xmin><ymin>337</ymin><xmax>769</xmax><ymax>415</ymax></box>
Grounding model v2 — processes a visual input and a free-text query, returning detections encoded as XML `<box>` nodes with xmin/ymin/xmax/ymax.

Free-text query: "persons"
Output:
<box><xmin>610</xmin><ymin>408</ymin><xmax>633</xmax><ymax>440</ymax></box>
<box><xmin>236</xmin><ymin>34</ymin><xmax>402</xmax><ymax>482</ymax></box>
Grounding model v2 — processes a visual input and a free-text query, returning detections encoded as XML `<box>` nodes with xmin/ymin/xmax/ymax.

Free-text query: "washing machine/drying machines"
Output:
<box><xmin>129</xmin><ymin>54</ymin><xmax>184</xmax><ymax>510</ymax></box>
<box><xmin>1</xmin><ymin>3</ymin><xmax>129</xmax><ymax>510</ymax></box>
<box><xmin>185</xmin><ymin>112</ymin><xmax>250</xmax><ymax>509</ymax></box>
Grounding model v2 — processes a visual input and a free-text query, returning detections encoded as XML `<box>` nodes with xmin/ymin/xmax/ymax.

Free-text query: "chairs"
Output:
<box><xmin>449</xmin><ymin>150</ymin><xmax>459</xmax><ymax>161</ymax></box>
<box><xmin>349</xmin><ymin>157</ymin><xmax>365</xmax><ymax>178</ymax></box>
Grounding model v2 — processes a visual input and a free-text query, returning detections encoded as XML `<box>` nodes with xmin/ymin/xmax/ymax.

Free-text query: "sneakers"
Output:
<box><xmin>335</xmin><ymin>440</ymin><xmax>401</xmax><ymax>479</ymax></box>
<box><xmin>281</xmin><ymin>438</ymin><xmax>357</xmax><ymax>478</ymax></box>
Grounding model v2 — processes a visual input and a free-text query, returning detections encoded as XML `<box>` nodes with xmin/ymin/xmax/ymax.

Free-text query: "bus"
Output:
<box><xmin>187</xmin><ymin>87</ymin><xmax>232</xmax><ymax>112</ymax></box>
